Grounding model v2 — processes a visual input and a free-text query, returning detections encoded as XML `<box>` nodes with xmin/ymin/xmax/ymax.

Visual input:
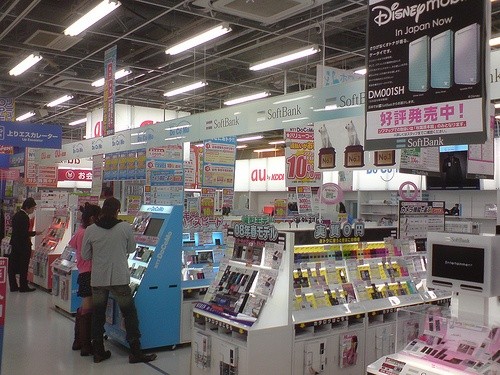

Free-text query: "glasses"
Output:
<box><xmin>116</xmin><ymin>208</ymin><xmax>121</xmax><ymax>214</ymax></box>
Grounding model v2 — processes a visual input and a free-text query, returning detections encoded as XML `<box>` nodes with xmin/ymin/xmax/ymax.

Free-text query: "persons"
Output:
<box><xmin>68</xmin><ymin>202</ymin><xmax>103</xmax><ymax>356</ymax></box>
<box><xmin>8</xmin><ymin>197</ymin><xmax>43</xmax><ymax>292</ymax></box>
<box><xmin>81</xmin><ymin>197</ymin><xmax>158</xmax><ymax>363</ymax></box>
<box><xmin>445</xmin><ymin>203</ymin><xmax>459</xmax><ymax>216</ymax></box>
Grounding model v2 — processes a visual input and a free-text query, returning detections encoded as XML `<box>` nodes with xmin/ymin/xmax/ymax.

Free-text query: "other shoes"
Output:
<box><xmin>19</xmin><ymin>286</ymin><xmax>36</xmax><ymax>292</ymax></box>
<box><xmin>130</xmin><ymin>353</ymin><xmax>157</xmax><ymax>363</ymax></box>
<box><xmin>10</xmin><ymin>287</ymin><xmax>20</xmax><ymax>292</ymax></box>
<box><xmin>94</xmin><ymin>350</ymin><xmax>112</xmax><ymax>362</ymax></box>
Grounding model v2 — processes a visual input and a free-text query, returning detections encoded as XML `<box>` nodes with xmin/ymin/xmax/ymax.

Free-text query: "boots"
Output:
<box><xmin>71</xmin><ymin>307</ymin><xmax>82</xmax><ymax>350</ymax></box>
<box><xmin>79</xmin><ymin>309</ymin><xmax>93</xmax><ymax>356</ymax></box>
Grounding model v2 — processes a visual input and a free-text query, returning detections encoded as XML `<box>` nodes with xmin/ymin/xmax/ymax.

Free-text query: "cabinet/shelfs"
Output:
<box><xmin>0</xmin><ymin>191</ymin><xmax>500</xmax><ymax>375</ymax></box>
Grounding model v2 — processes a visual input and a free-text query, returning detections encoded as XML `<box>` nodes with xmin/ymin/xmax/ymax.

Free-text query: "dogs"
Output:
<box><xmin>345</xmin><ymin>120</ymin><xmax>360</xmax><ymax>146</ymax></box>
<box><xmin>319</xmin><ymin>124</ymin><xmax>333</xmax><ymax>149</ymax></box>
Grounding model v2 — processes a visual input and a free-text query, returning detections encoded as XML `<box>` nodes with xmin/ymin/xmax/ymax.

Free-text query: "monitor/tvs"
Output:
<box><xmin>431</xmin><ymin>243</ymin><xmax>485</xmax><ymax>285</ymax></box>
<box><xmin>142</xmin><ymin>217</ymin><xmax>165</xmax><ymax>237</ymax></box>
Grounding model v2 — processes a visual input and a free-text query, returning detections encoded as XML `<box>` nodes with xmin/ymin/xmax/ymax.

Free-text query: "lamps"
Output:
<box><xmin>8</xmin><ymin>0</ymin><xmax>366</xmax><ymax>152</ymax></box>
